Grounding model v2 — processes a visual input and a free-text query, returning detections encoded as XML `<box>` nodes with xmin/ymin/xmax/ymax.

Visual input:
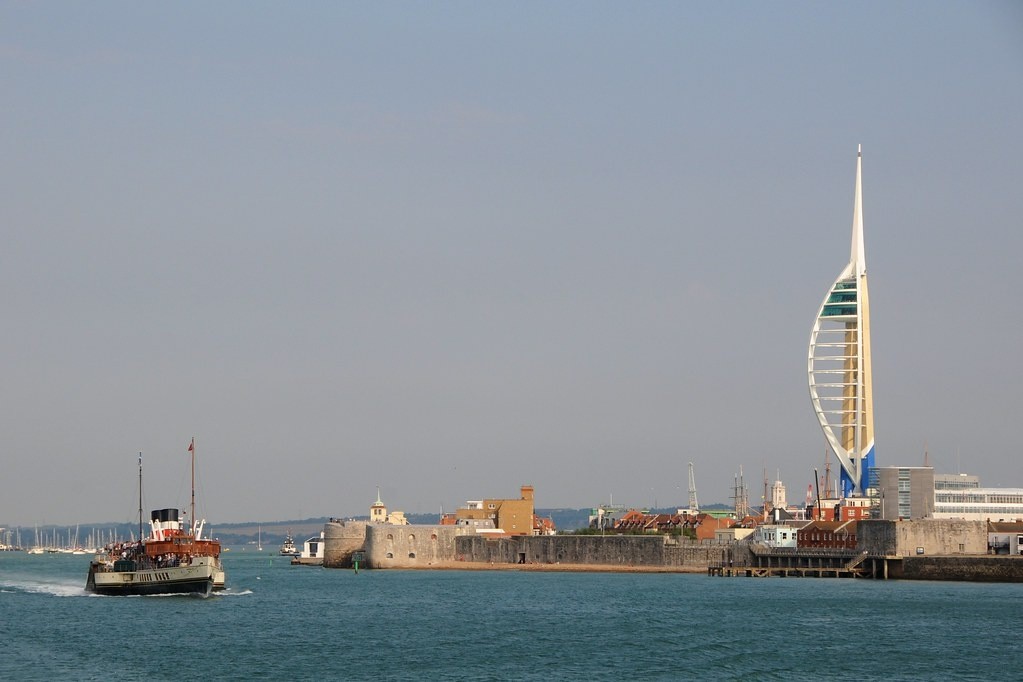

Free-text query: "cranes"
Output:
<box><xmin>687</xmin><ymin>461</ymin><xmax>699</xmax><ymax>512</ymax></box>
<box><xmin>730</xmin><ymin>450</ymin><xmax>836</xmax><ymax>524</ymax></box>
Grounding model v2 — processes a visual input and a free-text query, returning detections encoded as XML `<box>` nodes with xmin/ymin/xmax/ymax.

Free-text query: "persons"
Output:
<box><xmin>106</xmin><ymin>539</ymin><xmax>193</xmax><ymax>568</ymax></box>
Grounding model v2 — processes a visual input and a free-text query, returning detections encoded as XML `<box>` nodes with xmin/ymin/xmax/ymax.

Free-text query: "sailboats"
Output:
<box><xmin>0</xmin><ymin>521</ymin><xmax>140</xmax><ymax>555</ymax></box>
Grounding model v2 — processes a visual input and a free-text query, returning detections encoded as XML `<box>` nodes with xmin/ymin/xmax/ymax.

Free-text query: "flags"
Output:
<box><xmin>188</xmin><ymin>444</ymin><xmax>193</xmax><ymax>451</ymax></box>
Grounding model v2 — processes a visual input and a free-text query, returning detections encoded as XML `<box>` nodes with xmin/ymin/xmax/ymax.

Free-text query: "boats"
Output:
<box><xmin>279</xmin><ymin>527</ymin><xmax>300</xmax><ymax>556</ymax></box>
<box><xmin>83</xmin><ymin>435</ymin><xmax>225</xmax><ymax>594</ymax></box>
